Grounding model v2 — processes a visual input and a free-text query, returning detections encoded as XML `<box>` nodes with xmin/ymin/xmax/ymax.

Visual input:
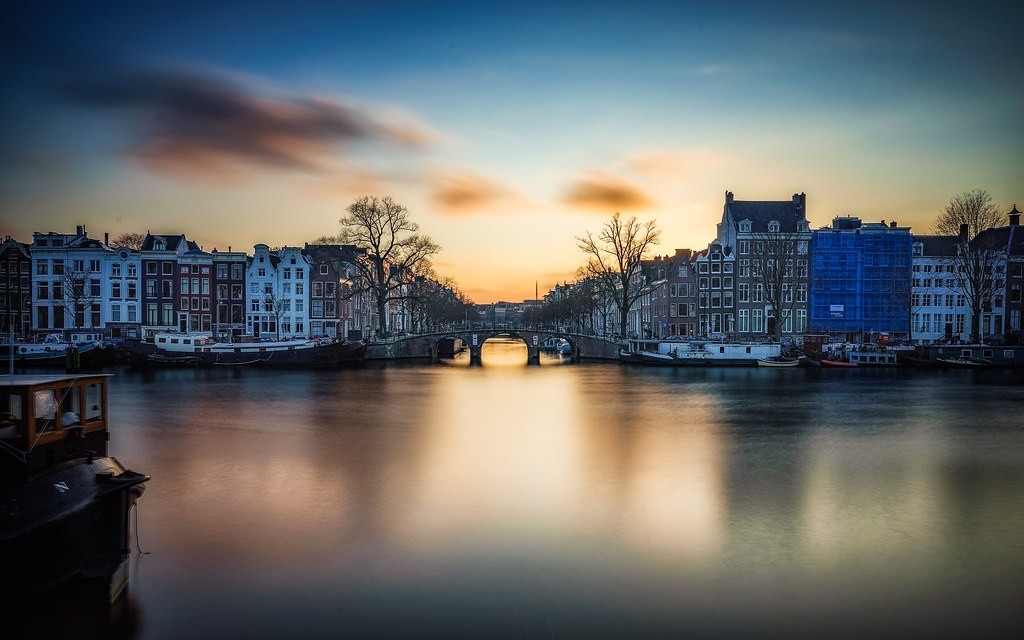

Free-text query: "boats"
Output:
<box><xmin>619</xmin><ymin>339</ymin><xmax>993</xmax><ymax>369</ymax></box>
<box><xmin>0</xmin><ymin>332</ymin><xmax>367</xmax><ymax>368</ymax></box>
<box><xmin>0</xmin><ymin>374</ymin><xmax>151</xmax><ymax>640</ymax></box>
